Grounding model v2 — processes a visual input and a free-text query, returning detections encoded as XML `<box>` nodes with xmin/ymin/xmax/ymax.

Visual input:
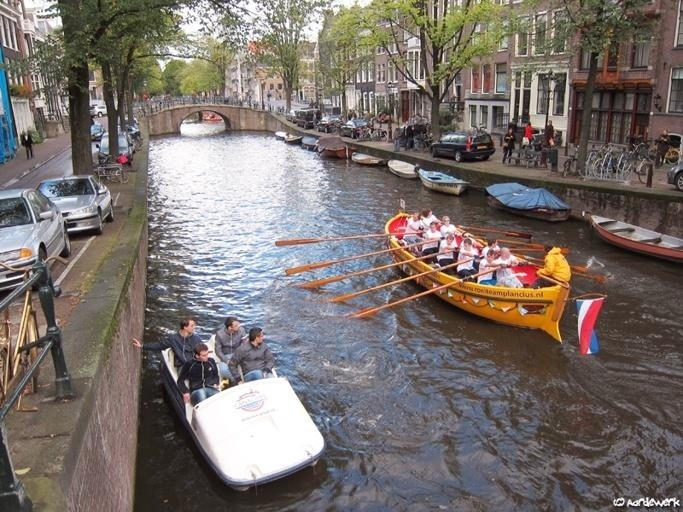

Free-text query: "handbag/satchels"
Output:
<box><xmin>522</xmin><ymin>137</ymin><xmax>529</xmax><ymax>146</ymax></box>
<box><xmin>503</xmin><ymin>143</ymin><xmax>508</xmax><ymax>146</ymax></box>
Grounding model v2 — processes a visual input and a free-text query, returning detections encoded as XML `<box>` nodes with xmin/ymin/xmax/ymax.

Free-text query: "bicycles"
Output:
<box><xmin>647</xmin><ymin>137</ymin><xmax>682</xmax><ymax>166</ymax></box>
<box><xmin>560</xmin><ymin>141</ymin><xmax>657</xmax><ymax>187</ymax></box>
<box><xmin>327</xmin><ymin>120</ymin><xmax>343</xmax><ymax>136</ymax></box>
<box><xmin>355</xmin><ymin>123</ymin><xmax>388</xmax><ymax>142</ymax></box>
<box><xmin>405</xmin><ymin>132</ymin><xmax>432</xmax><ymax>154</ymax></box>
<box><xmin>93</xmin><ymin>153</ymin><xmax>131</xmax><ymax>184</ymax></box>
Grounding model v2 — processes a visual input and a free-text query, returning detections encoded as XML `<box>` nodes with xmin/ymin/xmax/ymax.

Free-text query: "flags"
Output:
<box><xmin>573</xmin><ymin>298</ymin><xmax>604</xmax><ymax>355</ymax></box>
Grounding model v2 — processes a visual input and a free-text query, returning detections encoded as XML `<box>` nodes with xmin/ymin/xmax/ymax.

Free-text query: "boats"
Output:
<box><xmin>351</xmin><ymin>151</ymin><xmax>386</xmax><ymax>168</ymax></box>
<box><xmin>386</xmin><ymin>158</ymin><xmax>421</xmax><ymax>180</ymax></box>
<box><xmin>587</xmin><ymin>214</ymin><xmax>682</xmax><ymax>263</ymax></box>
<box><xmin>483</xmin><ymin>181</ymin><xmax>572</xmax><ymax>223</ymax></box>
<box><xmin>417</xmin><ymin>170</ymin><xmax>471</xmax><ymax>196</ymax></box>
<box><xmin>314</xmin><ymin>136</ymin><xmax>351</xmax><ymax>160</ymax></box>
<box><xmin>382</xmin><ymin>207</ymin><xmax>571</xmax><ymax>345</ymax></box>
<box><xmin>272</xmin><ymin>129</ymin><xmax>317</xmax><ymax>151</ymax></box>
<box><xmin>158</xmin><ymin>332</ymin><xmax>327</xmax><ymax>492</ymax></box>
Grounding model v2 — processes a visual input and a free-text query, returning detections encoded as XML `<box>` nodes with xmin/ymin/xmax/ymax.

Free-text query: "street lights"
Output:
<box><xmin>540</xmin><ymin>67</ymin><xmax>559</xmax><ymax>149</ymax></box>
<box><xmin>385</xmin><ymin>81</ymin><xmax>395</xmax><ymax>142</ymax></box>
<box><xmin>143</xmin><ymin>80</ymin><xmax>147</xmax><ymax>105</ymax></box>
<box><xmin>258</xmin><ymin>77</ymin><xmax>265</xmax><ymax>111</ymax></box>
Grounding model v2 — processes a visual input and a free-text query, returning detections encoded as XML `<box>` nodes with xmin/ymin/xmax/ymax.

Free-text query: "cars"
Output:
<box><xmin>33</xmin><ymin>174</ymin><xmax>114</xmax><ymax>235</ymax></box>
<box><xmin>90</xmin><ymin>121</ymin><xmax>105</xmax><ymax>142</ymax></box>
<box><xmin>0</xmin><ymin>185</ymin><xmax>70</xmax><ymax>293</ymax></box>
<box><xmin>115</xmin><ymin>116</ymin><xmax>141</xmax><ymax>140</ymax></box>
<box><xmin>665</xmin><ymin>165</ymin><xmax>682</xmax><ymax>192</ymax></box>
<box><xmin>340</xmin><ymin>118</ymin><xmax>372</xmax><ymax>140</ymax></box>
<box><xmin>315</xmin><ymin>117</ymin><xmax>342</xmax><ymax>133</ymax></box>
<box><xmin>89</xmin><ymin>105</ymin><xmax>107</xmax><ymax>117</ymax></box>
<box><xmin>400</xmin><ymin>123</ymin><xmax>426</xmax><ymax>134</ymax></box>
<box><xmin>285</xmin><ymin>109</ymin><xmax>299</xmax><ymax>124</ymax></box>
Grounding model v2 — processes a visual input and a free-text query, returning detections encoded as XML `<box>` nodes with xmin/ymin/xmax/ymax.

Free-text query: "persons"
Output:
<box><xmin>501</xmin><ymin>128</ymin><xmax>516</xmax><ymax>164</ymax></box>
<box><xmin>346</xmin><ymin>108</ymin><xmax>358</xmax><ymax>122</ymax></box>
<box><xmin>508</xmin><ymin>118</ymin><xmax>517</xmax><ymax>134</ymax></box>
<box><xmin>655</xmin><ymin>130</ymin><xmax>670</xmax><ymax>170</ymax></box>
<box><xmin>214</xmin><ymin>317</ymin><xmax>247</xmax><ymax>382</ymax></box>
<box><xmin>403</xmin><ymin>209</ymin><xmax>523</xmax><ymax>288</ymax></box>
<box><xmin>545</xmin><ymin>119</ymin><xmax>555</xmax><ymax>146</ymax></box>
<box><xmin>530</xmin><ymin>245</ymin><xmax>570</xmax><ymax>288</ymax></box>
<box><xmin>177</xmin><ymin>345</ymin><xmax>220</xmax><ymax>405</ymax></box>
<box><xmin>130</xmin><ymin>318</ymin><xmax>208</xmax><ymax>373</ymax></box>
<box><xmin>393</xmin><ymin>127</ymin><xmax>402</xmax><ymax>153</ymax></box>
<box><xmin>229</xmin><ymin>327</ymin><xmax>275</xmax><ymax>383</ymax></box>
<box><xmin>523</xmin><ymin>123</ymin><xmax>534</xmax><ymax>143</ymax></box>
<box><xmin>405</xmin><ymin>123</ymin><xmax>415</xmax><ymax>151</ymax></box>
<box><xmin>21</xmin><ymin>131</ymin><xmax>34</xmax><ymax>159</ymax></box>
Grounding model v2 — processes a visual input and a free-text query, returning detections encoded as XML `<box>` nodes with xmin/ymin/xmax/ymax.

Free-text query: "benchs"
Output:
<box><xmin>501</xmin><ymin>143</ymin><xmax>551</xmax><ymax>169</ymax></box>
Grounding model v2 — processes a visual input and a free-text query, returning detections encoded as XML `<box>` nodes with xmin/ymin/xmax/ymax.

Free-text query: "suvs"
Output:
<box><xmin>430</xmin><ymin>130</ymin><xmax>496</xmax><ymax>161</ymax></box>
<box><xmin>295</xmin><ymin>110</ymin><xmax>321</xmax><ymax>129</ymax></box>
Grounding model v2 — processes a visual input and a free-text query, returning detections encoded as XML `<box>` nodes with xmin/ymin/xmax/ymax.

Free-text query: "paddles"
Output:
<box><xmin>331</xmin><ymin>258</ymin><xmax>474</xmax><ymax>302</ymax></box>
<box><xmin>298</xmin><ymin>248</ymin><xmax>454</xmax><ymax>289</ymax></box>
<box><xmin>528</xmin><ymin>261</ymin><xmax>606</xmax><ymax>284</ymax></box>
<box><xmin>496</xmin><ymin>238</ymin><xmax>545</xmax><ymax>249</ymax></box>
<box><xmin>285</xmin><ymin>238</ymin><xmax>440</xmax><ymax>275</ymax></box>
<box><xmin>460</xmin><ymin>225</ymin><xmax>532</xmax><ymax>239</ymax></box>
<box><xmin>515</xmin><ymin>252</ymin><xmax>589</xmax><ymax>272</ymax></box>
<box><xmin>510</xmin><ymin>245</ymin><xmax>569</xmax><ymax>254</ymax></box>
<box><xmin>275</xmin><ymin>230</ymin><xmax>426</xmax><ymax>246</ymax></box>
<box><xmin>350</xmin><ymin>266</ymin><xmax>500</xmax><ymax>318</ymax></box>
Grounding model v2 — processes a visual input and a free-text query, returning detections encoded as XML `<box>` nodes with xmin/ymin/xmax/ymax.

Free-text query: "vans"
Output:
<box><xmin>95</xmin><ymin>132</ymin><xmax>134</xmax><ymax>167</ymax></box>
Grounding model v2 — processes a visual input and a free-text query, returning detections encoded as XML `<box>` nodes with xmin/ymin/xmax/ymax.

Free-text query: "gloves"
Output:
<box><xmin>474</xmin><ymin>255</ymin><xmax>484</xmax><ymax>259</ymax></box>
<box><xmin>499</xmin><ymin>260</ymin><xmax>529</xmax><ymax>268</ymax></box>
